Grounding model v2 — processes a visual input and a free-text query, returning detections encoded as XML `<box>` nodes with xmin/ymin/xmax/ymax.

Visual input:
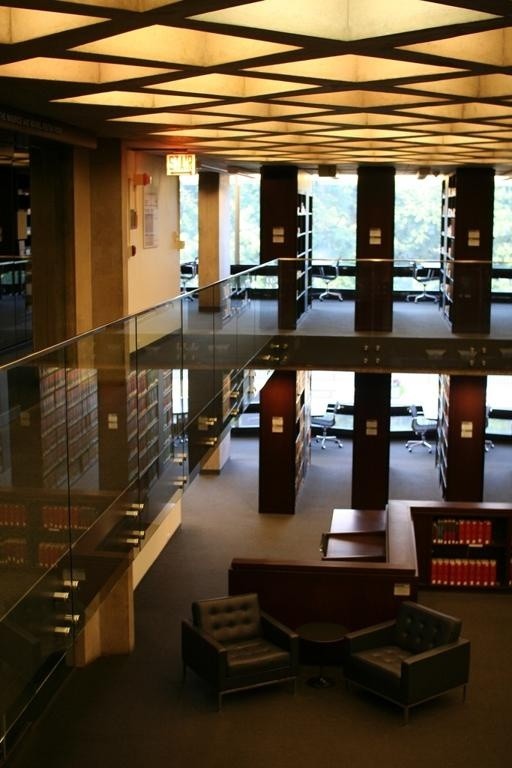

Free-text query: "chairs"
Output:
<box><xmin>182</xmin><ymin>252</ymin><xmax>441</xmax><ymax>309</ymax></box>
<box><xmin>180</xmin><ymin>595</ymin><xmax>301</xmax><ymax>713</ymax></box>
<box><xmin>314</xmin><ymin>400</ymin><xmax>494</xmax><ymax>454</ymax></box>
<box><xmin>343</xmin><ymin>601</ymin><xmax>478</xmax><ymax>722</ymax></box>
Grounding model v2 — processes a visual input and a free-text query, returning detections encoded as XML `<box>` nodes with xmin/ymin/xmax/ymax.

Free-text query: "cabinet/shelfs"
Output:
<box><xmin>11</xmin><ymin>364</ymin><xmax>98</xmax><ymax>487</ymax></box>
<box><xmin>441</xmin><ymin>167</ymin><xmax>495</xmax><ymax>334</ymax></box>
<box><xmin>0</xmin><ymin>488</ymin><xmax>119</xmax><ymax>575</ymax></box>
<box><xmin>258</xmin><ymin>365</ymin><xmax>312</xmax><ymax>515</ymax></box>
<box><xmin>434</xmin><ymin>373</ymin><xmax>484</xmax><ymax>502</ymax></box>
<box><xmin>258</xmin><ymin>163</ymin><xmax>314</xmax><ymax>333</ymax></box>
<box><xmin>423</xmin><ymin>510</ymin><xmax>510</xmax><ymax>589</ymax></box>
<box><xmin>99</xmin><ymin>368</ymin><xmax>174</xmax><ymax>499</ymax></box>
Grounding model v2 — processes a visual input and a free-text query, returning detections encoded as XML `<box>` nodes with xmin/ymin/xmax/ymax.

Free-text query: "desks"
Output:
<box><xmin>297</xmin><ymin>621</ymin><xmax>348</xmax><ymax>690</ymax></box>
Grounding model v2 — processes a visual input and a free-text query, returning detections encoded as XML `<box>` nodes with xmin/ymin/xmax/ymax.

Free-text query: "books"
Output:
<box><xmin>43</xmin><ymin>506</ymin><xmax>95</xmax><ymax>530</ymax></box>
<box><xmin>433</xmin><ymin>519</ymin><xmax>492</xmax><ymax>546</ymax></box>
<box><xmin>0</xmin><ymin>504</ymin><xmax>31</xmax><ymax>527</ymax></box>
<box><xmin>37</xmin><ymin>541</ymin><xmax>68</xmax><ymax>567</ymax></box>
<box><xmin>1</xmin><ymin>537</ymin><xmax>31</xmax><ymax>568</ymax></box>
<box><xmin>431</xmin><ymin>557</ymin><xmax>497</xmax><ymax>587</ymax></box>
